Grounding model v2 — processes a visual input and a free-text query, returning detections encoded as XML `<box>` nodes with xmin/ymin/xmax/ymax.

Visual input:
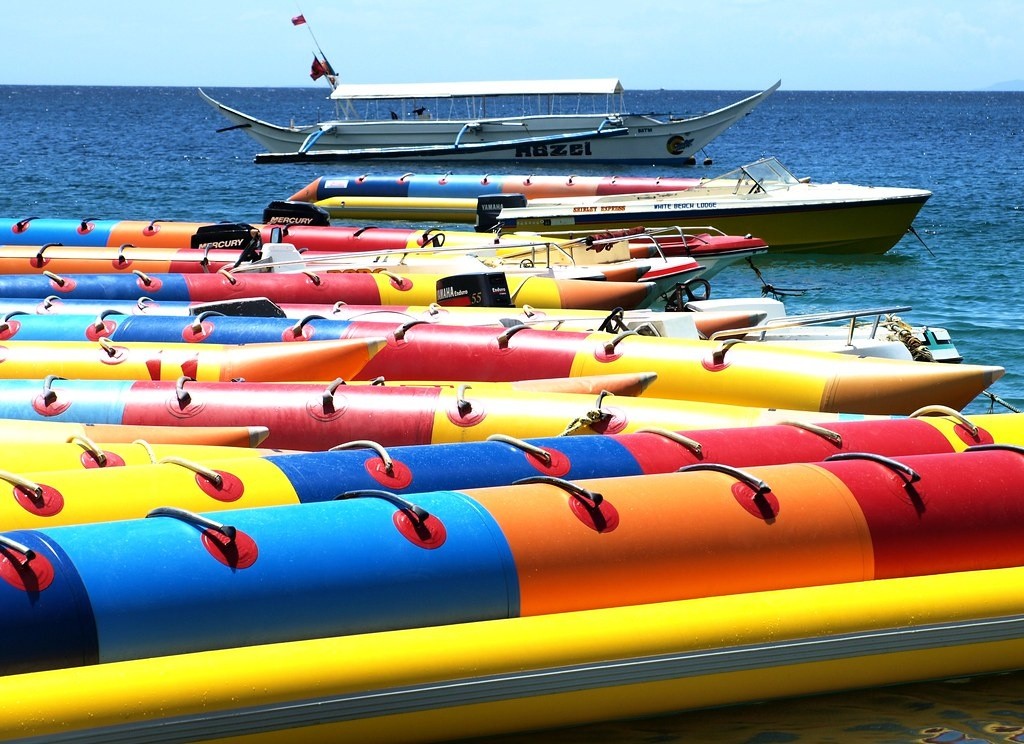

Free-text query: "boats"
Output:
<box><xmin>195</xmin><ymin>2</ymin><xmax>785</xmax><ymax>166</ymax></box>
<box><xmin>0</xmin><ymin>157</ymin><xmax>1023</xmax><ymax>744</ymax></box>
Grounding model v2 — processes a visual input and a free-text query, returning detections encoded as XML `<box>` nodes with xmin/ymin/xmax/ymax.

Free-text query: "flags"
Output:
<box><xmin>292</xmin><ymin>14</ymin><xmax>306</xmax><ymax>25</ymax></box>
<box><xmin>310</xmin><ymin>57</ymin><xmax>331</xmax><ymax>81</ymax></box>
